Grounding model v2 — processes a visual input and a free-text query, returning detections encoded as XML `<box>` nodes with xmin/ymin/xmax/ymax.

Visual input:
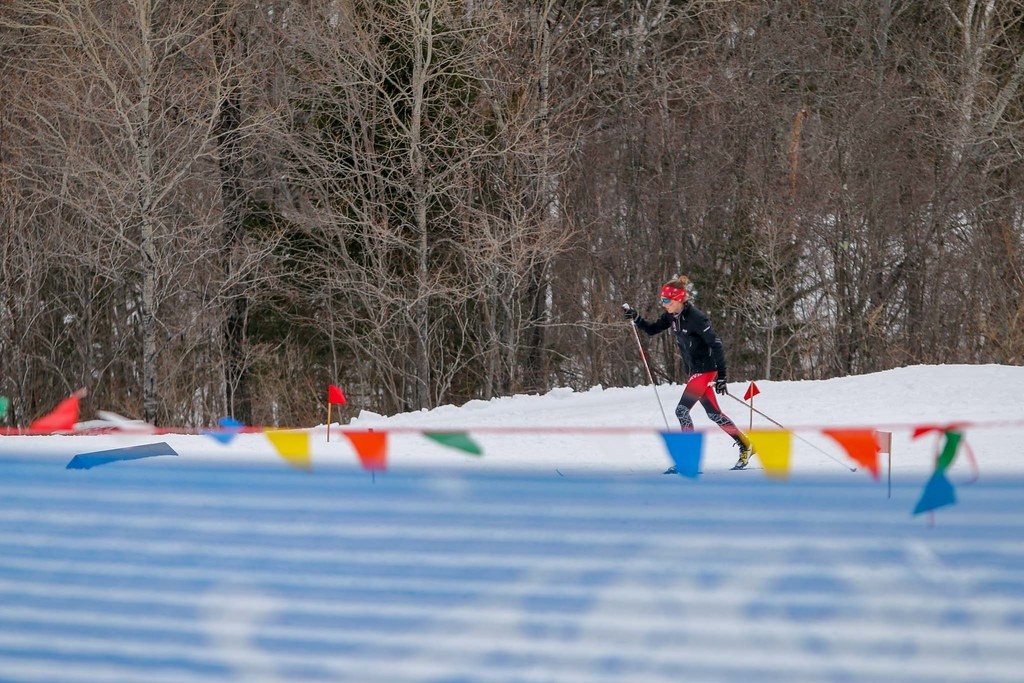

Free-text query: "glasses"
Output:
<box><xmin>660</xmin><ymin>298</ymin><xmax>670</xmax><ymax>303</ymax></box>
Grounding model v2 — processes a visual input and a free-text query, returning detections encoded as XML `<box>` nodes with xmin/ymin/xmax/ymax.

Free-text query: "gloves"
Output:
<box><xmin>621</xmin><ymin>305</ymin><xmax>638</xmax><ymax>320</ymax></box>
<box><xmin>715</xmin><ymin>379</ymin><xmax>728</xmax><ymax>395</ymax></box>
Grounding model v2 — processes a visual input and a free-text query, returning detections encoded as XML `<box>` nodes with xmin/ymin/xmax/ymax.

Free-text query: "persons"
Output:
<box><xmin>624</xmin><ymin>276</ymin><xmax>757</xmax><ymax>473</ymax></box>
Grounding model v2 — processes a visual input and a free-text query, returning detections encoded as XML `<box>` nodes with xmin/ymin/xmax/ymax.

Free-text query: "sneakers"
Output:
<box><xmin>735</xmin><ymin>443</ymin><xmax>755</xmax><ymax>468</ymax></box>
<box><xmin>668</xmin><ymin>464</ymin><xmax>679</xmax><ymax>474</ymax></box>
